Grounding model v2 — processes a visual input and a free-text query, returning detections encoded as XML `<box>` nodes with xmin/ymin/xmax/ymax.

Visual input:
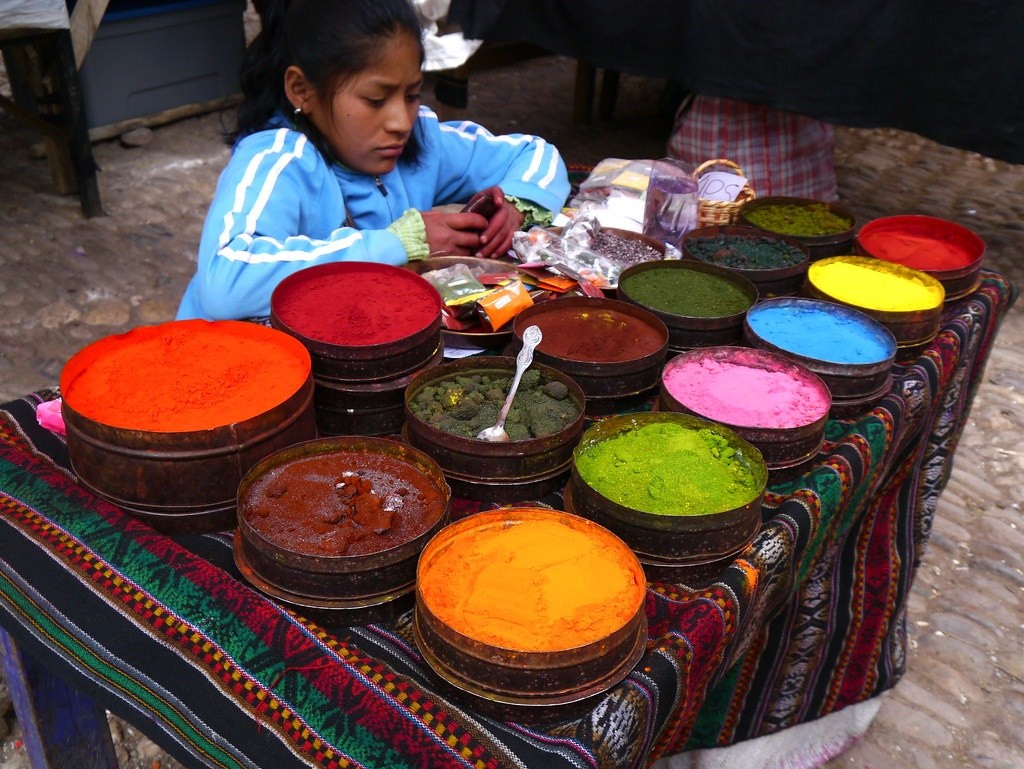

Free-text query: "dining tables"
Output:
<box><xmin>0</xmin><ymin>224</ymin><xmax>1020</xmax><ymax>769</ymax></box>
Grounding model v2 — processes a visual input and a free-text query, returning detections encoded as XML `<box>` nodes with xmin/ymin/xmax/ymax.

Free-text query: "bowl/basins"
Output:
<box><xmin>56</xmin><ymin>196</ymin><xmax>989</xmax><ymax>710</ymax></box>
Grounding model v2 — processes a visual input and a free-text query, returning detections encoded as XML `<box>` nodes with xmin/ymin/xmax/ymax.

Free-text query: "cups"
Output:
<box><xmin>642</xmin><ymin>156</ymin><xmax>700</xmax><ymax>260</ymax></box>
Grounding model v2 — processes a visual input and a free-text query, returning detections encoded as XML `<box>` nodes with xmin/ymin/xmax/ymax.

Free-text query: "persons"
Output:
<box><xmin>172</xmin><ymin>0</ymin><xmax>574</xmax><ymax>322</ymax></box>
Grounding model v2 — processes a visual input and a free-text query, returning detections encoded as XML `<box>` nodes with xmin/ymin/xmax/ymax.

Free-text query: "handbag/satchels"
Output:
<box><xmin>663</xmin><ymin>92</ymin><xmax>843</xmax><ymax>204</ymax></box>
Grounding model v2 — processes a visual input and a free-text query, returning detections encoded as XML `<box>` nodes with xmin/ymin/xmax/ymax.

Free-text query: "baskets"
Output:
<box><xmin>690</xmin><ymin>157</ymin><xmax>757</xmax><ymax>229</ymax></box>
